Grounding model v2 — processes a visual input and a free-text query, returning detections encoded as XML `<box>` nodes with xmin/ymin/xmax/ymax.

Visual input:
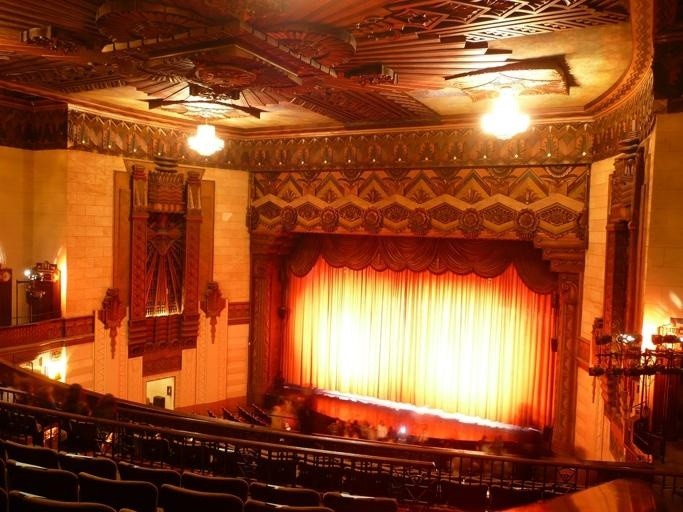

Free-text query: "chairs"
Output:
<box><xmin>0</xmin><ymin>401</ymin><xmax>537</xmax><ymax>512</ymax></box>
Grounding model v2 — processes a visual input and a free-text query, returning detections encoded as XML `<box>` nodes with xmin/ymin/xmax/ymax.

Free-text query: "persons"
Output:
<box><xmin>359</xmin><ymin>419</ymin><xmax>370</xmax><ymax>438</ymax></box>
<box><xmin>374</xmin><ymin>418</ymin><xmax>387</xmax><ymax>438</ymax></box>
<box><xmin>386</xmin><ymin>425</ymin><xmax>396</xmax><ymax>438</ymax></box>
<box><xmin>333</xmin><ymin>417</ymin><xmax>345</xmax><ymax>436</ymax></box>
<box><xmin>11</xmin><ymin>376</ymin><xmax>123</xmax><ymax>456</ymax></box>
<box><xmin>351</xmin><ymin>419</ymin><xmax>361</xmax><ymax>438</ymax></box>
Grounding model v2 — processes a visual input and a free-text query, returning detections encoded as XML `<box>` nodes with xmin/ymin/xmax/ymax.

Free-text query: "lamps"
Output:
<box><xmin>187</xmin><ymin>117</ymin><xmax>224</xmax><ymax>156</ymax></box>
<box><xmin>480</xmin><ymin>85</ymin><xmax>529</xmax><ymax>138</ymax></box>
